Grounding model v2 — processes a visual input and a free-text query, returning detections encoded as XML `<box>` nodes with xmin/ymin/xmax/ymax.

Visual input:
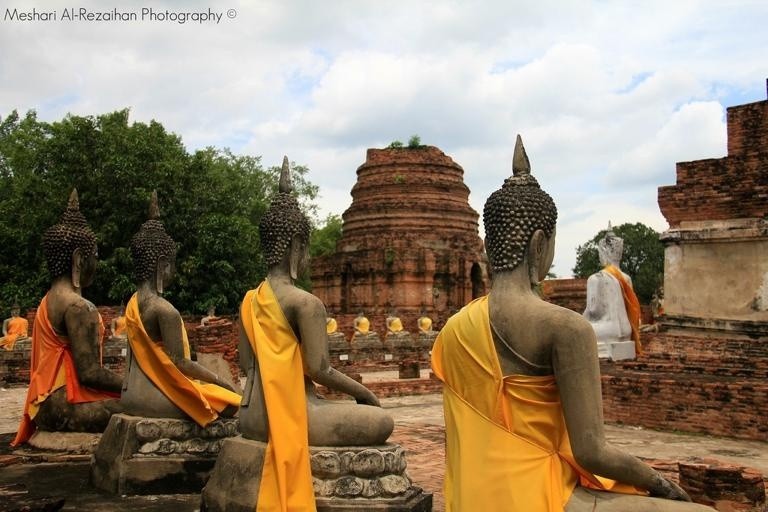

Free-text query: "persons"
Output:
<box><xmin>24</xmin><ymin>186</ymin><xmax>125</xmax><ymax>430</ymax></box>
<box><xmin>237</xmin><ymin>153</ymin><xmax>396</xmax><ymax>446</ymax></box>
<box><xmin>430</xmin><ymin>135</ymin><xmax>717</xmax><ymax>512</ymax></box>
<box><xmin>583</xmin><ymin>220</ymin><xmax>645</xmax><ymax>357</ymax></box>
<box><xmin>121</xmin><ymin>189</ymin><xmax>241</xmax><ymax>428</ymax></box>
<box><xmin>1</xmin><ymin>305</ymin><xmax>436</xmax><ymax>353</ymax></box>
